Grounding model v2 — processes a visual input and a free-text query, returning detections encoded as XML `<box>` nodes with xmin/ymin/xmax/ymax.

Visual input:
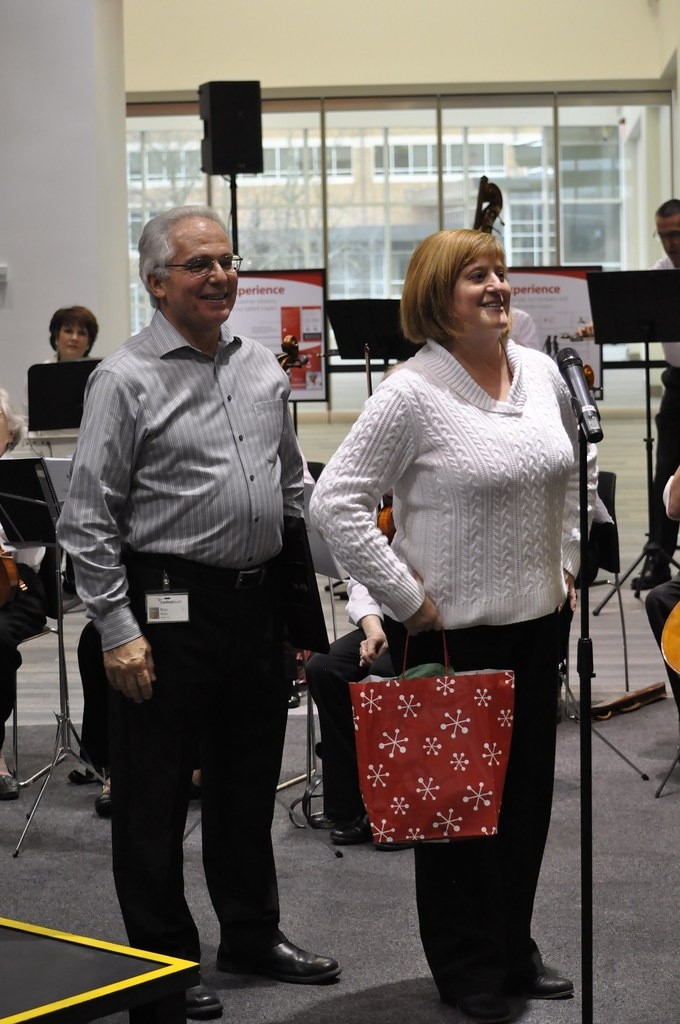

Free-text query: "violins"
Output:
<box><xmin>278</xmin><ymin>335</ymin><xmax>308</xmax><ymax>371</ymax></box>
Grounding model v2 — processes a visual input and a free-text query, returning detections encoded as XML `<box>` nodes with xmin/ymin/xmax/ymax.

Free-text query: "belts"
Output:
<box><xmin>132</xmin><ymin>549</ymin><xmax>298</xmax><ymax>589</ymax></box>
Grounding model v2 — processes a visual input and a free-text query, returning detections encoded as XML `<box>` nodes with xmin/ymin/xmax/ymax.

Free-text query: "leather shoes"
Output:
<box><xmin>184</xmin><ymin>975</ymin><xmax>224</xmax><ymax>1018</ymax></box>
<box><xmin>216</xmin><ymin>928</ymin><xmax>341</xmax><ymax>984</ymax></box>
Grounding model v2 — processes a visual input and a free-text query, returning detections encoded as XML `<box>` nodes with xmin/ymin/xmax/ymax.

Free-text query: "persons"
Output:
<box><xmin>631</xmin><ymin>198</ymin><xmax>680</xmax><ymax>592</ymax></box>
<box><xmin>644</xmin><ymin>467</ymin><xmax>680</xmax><ymax>713</ymax></box>
<box><xmin>49</xmin><ymin>306</ymin><xmax>99</xmax><ymax>362</ymax></box>
<box><xmin>0</xmin><ymin>389</ymin><xmax>48</xmax><ymax>801</ymax></box>
<box><xmin>304</xmin><ymin>573</ymin><xmax>413</xmax><ymax>849</ymax></box>
<box><xmin>75</xmin><ymin>622</ymin><xmax>202</xmax><ymax>815</ymax></box>
<box><xmin>55</xmin><ymin>205</ymin><xmax>341</xmax><ymax>1014</ymax></box>
<box><xmin>309</xmin><ymin>231</ymin><xmax>599</xmax><ymax>1021</ymax></box>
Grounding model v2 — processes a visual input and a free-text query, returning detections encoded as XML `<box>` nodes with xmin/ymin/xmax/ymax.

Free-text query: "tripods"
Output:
<box><xmin>585</xmin><ymin>267</ymin><xmax>680</xmax><ymax>616</ymax></box>
<box><xmin>0</xmin><ymin>457</ymin><xmax>110</xmax><ymax>856</ymax></box>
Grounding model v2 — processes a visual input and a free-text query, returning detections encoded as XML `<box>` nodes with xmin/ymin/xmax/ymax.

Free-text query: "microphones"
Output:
<box><xmin>556</xmin><ymin>347</ymin><xmax>604</xmax><ymax>444</ymax></box>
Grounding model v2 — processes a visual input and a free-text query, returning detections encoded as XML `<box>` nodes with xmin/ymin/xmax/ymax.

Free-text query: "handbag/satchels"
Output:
<box><xmin>348</xmin><ymin>628</ymin><xmax>516</xmax><ymax>847</ymax></box>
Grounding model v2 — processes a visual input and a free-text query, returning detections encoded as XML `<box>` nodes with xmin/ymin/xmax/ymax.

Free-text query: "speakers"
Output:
<box><xmin>197</xmin><ymin>81</ymin><xmax>264</xmax><ymax>175</ymax></box>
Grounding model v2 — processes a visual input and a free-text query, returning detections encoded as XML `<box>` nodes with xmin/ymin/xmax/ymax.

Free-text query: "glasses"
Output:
<box><xmin>164</xmin><ymin>254</ymin><xmax>243</xmax><ymax>278</ymax></box>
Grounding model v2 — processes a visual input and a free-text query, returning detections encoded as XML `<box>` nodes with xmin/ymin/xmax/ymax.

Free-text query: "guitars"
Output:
<box><xmin>659</xmin><ymin>598</ymin><xmax>680</xmax><ymax>672</ymax></box>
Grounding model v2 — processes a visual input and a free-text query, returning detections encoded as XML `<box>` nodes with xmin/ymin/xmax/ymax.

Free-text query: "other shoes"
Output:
<box><xmin>330</xmin><ymin>806</ymin><xmax>414</xmax><ymax>851</ymax></box>
<box><xmin>0</xmin><ymin>761</ymin><xmax>19</xmax><ymax>799</ymax></box>
<box><xmin>94</xmin><ymin>776</ymin><xmax>111</xmax><ymax>814</ymax></box>
<box><xmin>631</xmin><ymin>559</ymin><xmax>671</xmax><ymax>588</ymax></box>
